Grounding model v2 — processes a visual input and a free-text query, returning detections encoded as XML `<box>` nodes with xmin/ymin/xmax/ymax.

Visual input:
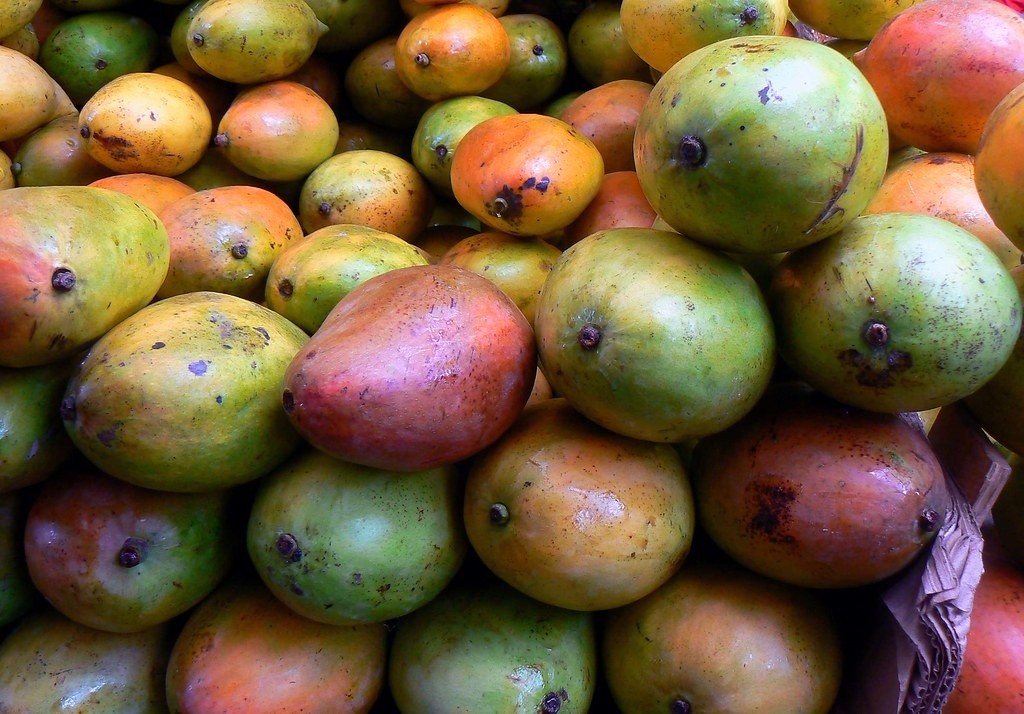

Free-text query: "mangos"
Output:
<box><xmin>0</xmin><ymin>0</ymin><xmax>1024</xmax><ymax>714</ymax></box>
